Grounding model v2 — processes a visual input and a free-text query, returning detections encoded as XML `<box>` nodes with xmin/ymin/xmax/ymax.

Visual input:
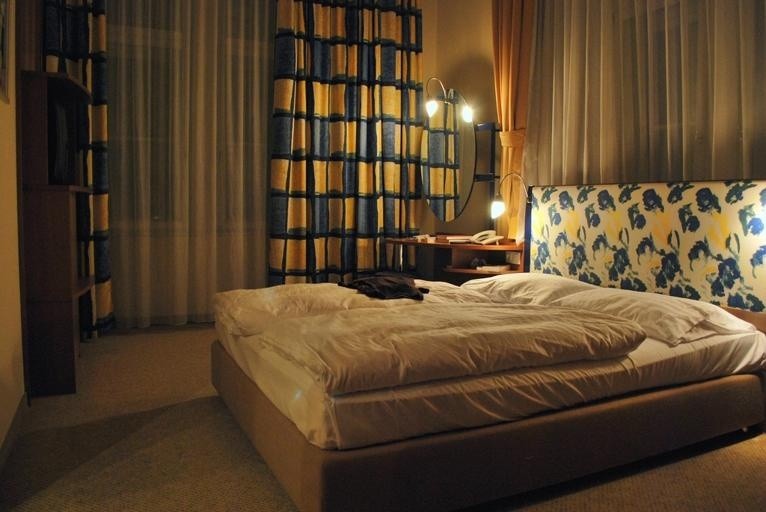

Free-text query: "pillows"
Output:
<box><xmin>462</xmin><ymin>270</ymin><xmax>605</xmax><ymax>306</ymax></box>
<box><xmin>548</xmin><ymin>286</ymin><xmax>757</xmax><ymax>347</ymax></box>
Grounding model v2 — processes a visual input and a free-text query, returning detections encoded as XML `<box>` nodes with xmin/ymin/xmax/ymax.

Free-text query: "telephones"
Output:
<box><xmin>471</xmin><ymin>230</ymin><xmax>504</xmax><ymax>244</ymax></box>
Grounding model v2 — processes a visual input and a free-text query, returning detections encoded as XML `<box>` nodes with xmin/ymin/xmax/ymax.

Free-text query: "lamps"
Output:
<box><xmin>425</xmin><ymin>75</ymin><xmax>455</xmax><ymax>118</ymax></box>
<box><xmin>491</xmin><ymin>172</ymin><xmax>531</xmax><ymax>219</ymax></box>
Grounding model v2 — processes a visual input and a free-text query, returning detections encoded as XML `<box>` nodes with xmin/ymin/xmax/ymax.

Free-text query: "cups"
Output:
<box><xmin>46</xmin><ymin>55</ymin><xmax>59</xmax><ymax>72</ymax></box>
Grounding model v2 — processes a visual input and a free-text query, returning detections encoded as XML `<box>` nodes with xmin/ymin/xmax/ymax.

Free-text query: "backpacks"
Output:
<box><xmin>340</xmin><ymin>276</ymin><xmax>429</xmax><ymax>300</ymax></box>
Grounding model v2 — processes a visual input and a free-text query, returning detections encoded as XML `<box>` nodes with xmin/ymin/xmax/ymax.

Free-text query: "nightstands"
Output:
<box><xmin>391</xmin><ymin>239</ymin><xmax>525</xmax><ymax>284</ymax></box>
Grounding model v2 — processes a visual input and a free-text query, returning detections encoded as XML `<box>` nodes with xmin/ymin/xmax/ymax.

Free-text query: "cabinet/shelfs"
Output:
<box><xmin>17</xmin><ymin>0</ymin><xmax>110</xmax><ymax>397</ymax></box>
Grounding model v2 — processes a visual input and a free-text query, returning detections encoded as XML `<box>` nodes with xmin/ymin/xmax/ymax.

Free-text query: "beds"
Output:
<box><xmin>210</xmin><ymin>178</ymin><xmax>766</xmax><ymax>512</ymax></box>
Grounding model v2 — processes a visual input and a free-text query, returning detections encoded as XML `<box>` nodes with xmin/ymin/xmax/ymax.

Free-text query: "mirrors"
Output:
<box><xmin>420</xmin><ymin>88</ymin><xmax>477</xmax><ymax>224</ymax></box>
